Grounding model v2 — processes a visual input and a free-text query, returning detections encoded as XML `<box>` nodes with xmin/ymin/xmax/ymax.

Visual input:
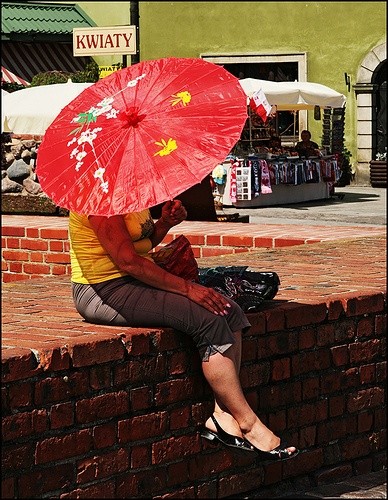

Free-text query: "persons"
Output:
<box><xmin>68</xmin><ymin>200</ymin><xmax>300</xmax><ymax>463</ymax></box>
<box><xmin>175</xmin><ymin>174</ymin><xmax>217</xmax><ymax>221</ymax></box>
<box><xmin>295</xmin><ymin>130</ymin><xmax>318</xmax><ymax>150</ymax></box>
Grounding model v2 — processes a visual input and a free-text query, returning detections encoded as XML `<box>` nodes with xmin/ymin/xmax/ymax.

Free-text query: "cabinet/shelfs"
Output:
<box><xmin>235</xmin><ymin>105</ymin><xmax>278</xmax><ymax>154</ymax></box>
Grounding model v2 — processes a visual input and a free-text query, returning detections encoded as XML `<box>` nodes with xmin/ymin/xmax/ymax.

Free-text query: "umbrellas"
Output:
<box><xmin>2</xmin><ymin>66</ymin><xmax>31</xmax><ymax>86</ymax></box>
<box><xmin>1</xmin><ymin>80</ymin><xmax>95</xmax><ymax>137</ymax></box>
<box><xmin>35</xmin><ymin>58</ymin><xmax>249</xmax><ymax>218</ymax></box>
<box><xmin>237</xmin><ymin>78</ymin><xmax>346</xmax><ymax>147</ymax></box>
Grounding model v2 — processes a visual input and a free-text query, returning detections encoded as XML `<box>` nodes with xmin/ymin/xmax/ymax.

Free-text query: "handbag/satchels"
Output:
<box><xmin>198</xmin><ymin>265</ymin><xmax>280</xmax><ymax>312</ymax></box>
<box><xmin>150</xmin><ymin>235</ymin><xmax>199</xmax><ymax>281</ymax></box>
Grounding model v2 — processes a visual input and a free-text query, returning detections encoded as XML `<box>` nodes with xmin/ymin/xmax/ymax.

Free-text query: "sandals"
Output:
<box><xmin>243</xmin><ymin>436</ymin><xmax>300</xmax><ymax>462</ymax></box>
<box><xmin>200</xmin><ymin>414</ymin><xmax>256</xmax><ymax>452</ymax></box>
